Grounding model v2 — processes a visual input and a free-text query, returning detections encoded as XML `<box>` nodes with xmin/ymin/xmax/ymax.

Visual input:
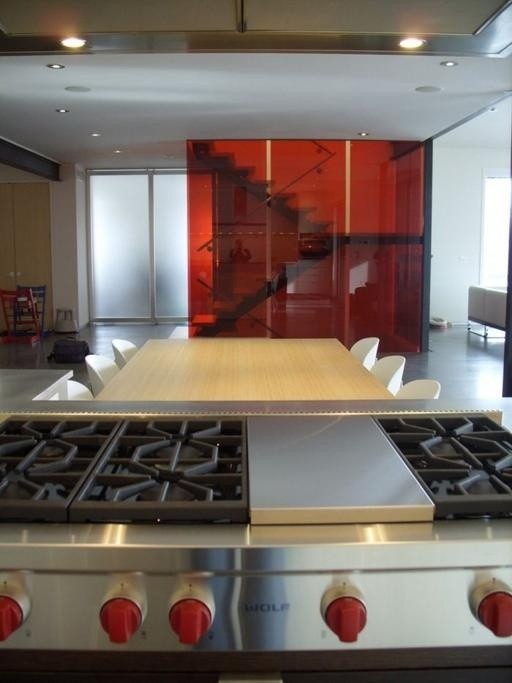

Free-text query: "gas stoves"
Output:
<box><xmin>0</xmin><ymin>411</ymin><xmax>511</xmax><ymax>664</ymax></box>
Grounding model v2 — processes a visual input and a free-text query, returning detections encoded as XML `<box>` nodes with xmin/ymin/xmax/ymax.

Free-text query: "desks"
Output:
<box><xmin>94</xmin><ymin>338</ymin><xmax>397</xmax><ymax>398</ymax></box>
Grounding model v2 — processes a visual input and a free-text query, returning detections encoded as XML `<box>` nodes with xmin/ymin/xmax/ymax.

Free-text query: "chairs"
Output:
<box><xmin>370</xmin><ymin>355</ymin><xmax>406</xmax><ymax>396</ymax></box>
<box><xmin>57</xmin><ymin>380</ymin><xmax>95</xmax><ymax>401</ymax></box>
<box><xmin>349</xmin><ymin>337</ymin><xmax>380</xmax><ymax>373</ymax></box>
<box><xmin>85</xmin><ymin>354</ymin><xmax>121</xmax><ymax>399</ymax></box>
<box><xmin>111</xmin><ymin>338</ymin><xmax>139</xmax><ymax>370</ymax></box>
<box><xmin>395</xmin><ymin>379</ymin><xmax>440</xmax><ymax>399</ymax></box>
<box><xmin>1</xmin><ymin>289</ymin><xmax>48</xmax><ymax>371</ymax></box>
